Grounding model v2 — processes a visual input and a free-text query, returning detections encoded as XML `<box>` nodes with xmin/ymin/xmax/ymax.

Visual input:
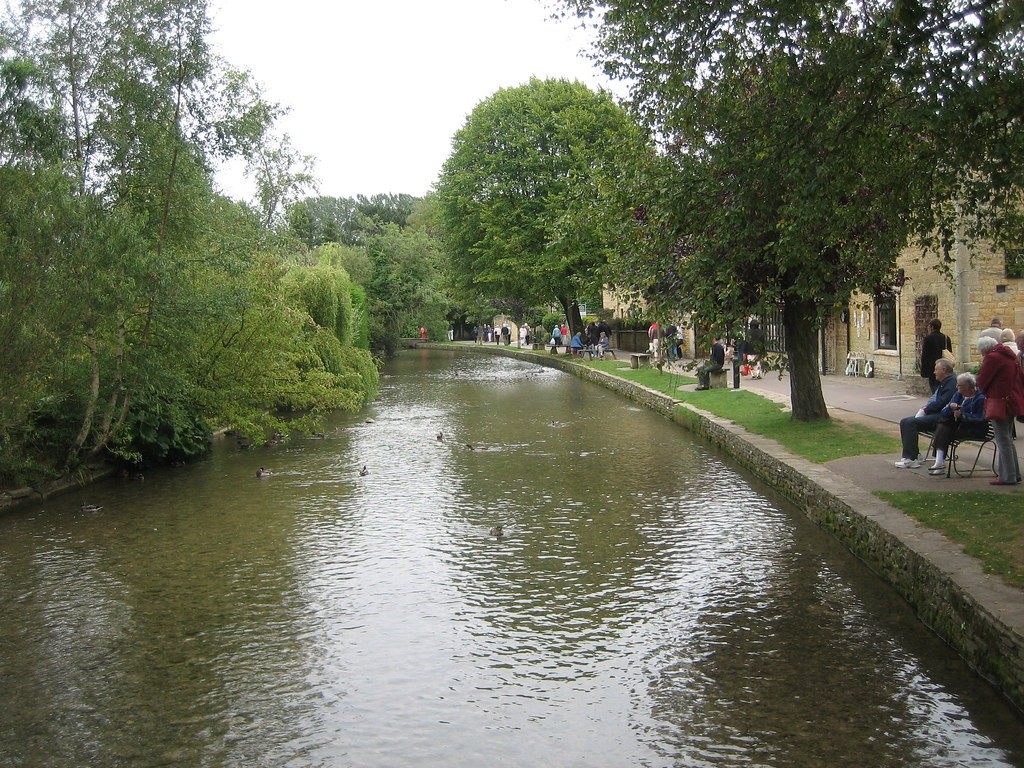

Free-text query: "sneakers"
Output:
<box><xmin>895</xmin><ymin>459</ymin><xmax>920</xmax><ymax>468</ymax></box>
<box><xmin>901</xmin><ymin>454</ymin><xmax>923</xmax><ymax>463</ymax></box>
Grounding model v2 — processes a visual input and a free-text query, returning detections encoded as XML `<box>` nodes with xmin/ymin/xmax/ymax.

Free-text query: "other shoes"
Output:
<box><xmin>757</xmin><ymin>375</ymin><xmax>762</xmax><ymax>379</ymax></box>
<box><xmin>1017</xmin><ymin>477</ymin><xmax>1022</xmax><ymax>484</ymax></box>
<box><xmin>990</xmin><ymin>478</ymin><xmax>1011</xmax><ymax>485</ymax></box>
<box><xmin>929</xmin><ymin>472</ymin><xmax>944</xmax><ymax>476</ymax></box>
<box><xmin>595</xmin><ymin>355</ymin><xmax>598</xmax><ymax>357</ymax></box>
<box><xmin>600</xmin><ymin>353</ymin><xmax>604</xmax><ymax>357</ymax></box>
<box><xmin>928</xmin><ymin>465</ymin><xmax>945</xmax><ymax>470</ymax></box>
<box><xmin>752</xmin><ymin>376</ymin><xmax>756</xmax><ymax>379</ymax></box>
<box><xmin>695</xmin><ymin>386</ymin><xmax>709</xmax><ymax>390</ymax></box>
<box><xmin>591</xmin><ymin>354</ymin><xmax>594</xmax><ymax>358</ymax></box>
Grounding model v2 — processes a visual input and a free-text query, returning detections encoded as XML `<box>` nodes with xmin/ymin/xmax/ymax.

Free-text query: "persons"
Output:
<box><xmin>649</xmin><ymin>321</ymin><xmax>684</xmax><ymax>361</ymax></box>
<box><xmin>746</xmin><ymin>320</ymin><xmax>766</xmax><ymax>380</ymax></box>
<box><xmin>695</xmin><ymin>335</ymin><xmax>725</xmax><ymax>390</ymax></box>
<box><xmin>895</xmin><ymin>318</ymin><xmax>1024</xmax><ymax>484</ymax></box>
<box><xmin>448</xmin><ymin>324</ymin><xmax>454</xmax><ymax>341</ymax></box>
<box><xmin>473</xmin><ymin>320</ymin><xmax>613</xmax><ymax>358</ymax></box>
<box><xmin>420</xmin><ymin>326</ymin><xmax>428</xmax><ymax>338</ymax></box>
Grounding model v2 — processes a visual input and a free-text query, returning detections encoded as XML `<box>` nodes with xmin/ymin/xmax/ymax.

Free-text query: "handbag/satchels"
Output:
<box><xmin>742</xmin><ymin>365</ymin><xmax>749</xmax><ymax>376</ymax></box>
<box><xmin>985</xmin><ymin>397</ymin><xmax>1008</xmax><ymax>418</ymax></box>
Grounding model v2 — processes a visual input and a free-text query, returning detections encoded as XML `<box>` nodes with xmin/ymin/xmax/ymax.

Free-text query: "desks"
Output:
<box><xmin>578</xmin><ymin>349</ymin><xmax>594</xmax><ymax>362</ymax></box>
<box><xmin>847</xmin><ymin>357</ymin><xmax>865</xmax><ymax>377</ymax></box>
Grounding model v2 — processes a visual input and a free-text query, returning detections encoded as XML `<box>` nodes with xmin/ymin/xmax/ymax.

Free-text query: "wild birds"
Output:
<box><xmin>80</xmin><ymin>364</ymin><xmax>569</xmax><ymax>536</ymax></box>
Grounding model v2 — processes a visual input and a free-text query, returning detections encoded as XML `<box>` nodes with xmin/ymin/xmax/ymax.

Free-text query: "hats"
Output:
<box><xmin>502</xmin><ymin>324</ymin><xmax>507</xmax><ymax>327</ymax></box>
<box><xmin>525</xmin><ymin>323</ymin><xmax>528</xmax><ymax>327</ymax></box>
<box><xmin>487</xmin><ymin>325</ymin><xmax>490</xmax><ymax>327</ymax></box>
<box><xmin>991</xmin><ymin>317</ymin><xmax>1003</xmax><ymax>325</ymax></box>
<box><xmin>555</xmin><ymin>325</ymin><xmax>558</xmax><ymax>327</ymax></box>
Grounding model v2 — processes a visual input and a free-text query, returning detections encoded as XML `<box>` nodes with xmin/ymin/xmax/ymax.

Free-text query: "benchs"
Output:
<box><xmin>919</xmin><ymin>420</ymin><xmax>999</xmax><ymax>479</ymax></box>
<box><xmin>630</xmin><ymin>354</ymin><xmax>651</xmax><ymax>369</ymax></box>
<box><xmin>710</xmin><ymin>368</ymin><xmax>731</xmax><ymax>388</ymax></box>
<box><xmin>604</xmin><ymin>348</ymin><xmax>616</xmax><ymax>360</ymax></box>
<box><xmin>546</xmin><ymin>344</ymin><xmax>570</xmax><ymax>354</ymax></box>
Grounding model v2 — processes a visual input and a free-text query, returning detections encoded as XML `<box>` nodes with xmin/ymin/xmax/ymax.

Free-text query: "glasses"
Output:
<box><xmin>956</xmin><ymin>384</ymin><xmax>964</xmax><ymax>387</ymax></box>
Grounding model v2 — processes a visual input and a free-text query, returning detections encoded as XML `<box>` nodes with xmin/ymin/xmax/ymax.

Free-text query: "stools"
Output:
<box><xmin>533</xmin><ymin>343</ymin><xmax>545</xmax><ymax>350</ymax></box>
